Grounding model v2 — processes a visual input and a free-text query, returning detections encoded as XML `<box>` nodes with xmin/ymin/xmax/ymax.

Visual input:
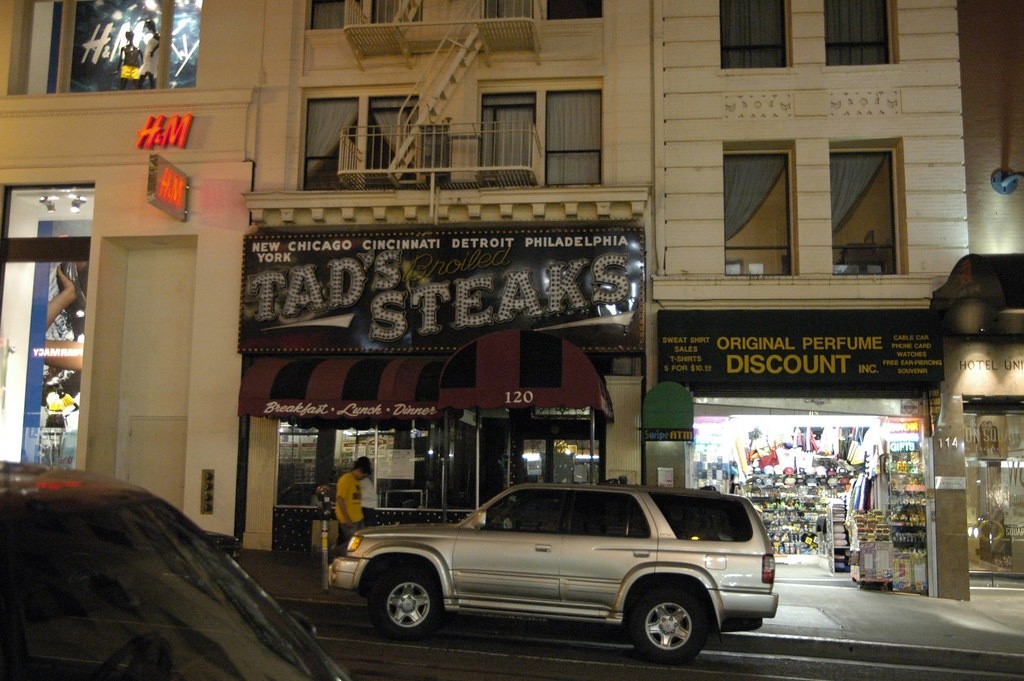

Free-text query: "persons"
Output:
<box><xmin>114</xmin><ymin>30</ymin><xmax>143</xmax><ymax>90</ymax></box>
<box><xmin>335</xmin><ymin>456</ymin><xmax>372</xmax><ymax>548</ymax></box>
<box><xmin>47</xmin><ymin>264</ymin><xmax>78</xmax><ymax>334</ymax></box>
<box><xmin>44</xmin><ymin>379</ymin><xmax>78</xmax><ymax>466</ymax></box>
<box><xmin>138</xmin><ymin>19</ymin><xmax>160</xmax><ymax>89</ymax></box>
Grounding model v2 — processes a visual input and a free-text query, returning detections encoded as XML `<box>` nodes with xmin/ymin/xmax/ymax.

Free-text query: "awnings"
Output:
<box><xmin>438</xmin><ymin>330</ymin><xmax>614</xmax><ymax>423</ymax></box>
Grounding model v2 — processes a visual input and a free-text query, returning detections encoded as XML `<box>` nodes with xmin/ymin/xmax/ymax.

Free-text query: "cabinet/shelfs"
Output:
<box><xmin>735</xmin><ymin>438</ymin><xmax>928</xmax><ymax>582</ymax></box>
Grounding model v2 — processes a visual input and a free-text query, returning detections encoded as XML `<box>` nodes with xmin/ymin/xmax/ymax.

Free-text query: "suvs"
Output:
<box><xmin>328</xmin><ymin>482</ymin><xmax>782</xmax><ymax>663</ymax></box>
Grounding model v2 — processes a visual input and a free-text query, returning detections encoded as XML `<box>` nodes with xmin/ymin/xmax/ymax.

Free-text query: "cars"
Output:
<box><xmin>1</xmin><ymin>458</ymin><xmax>352</xmax><ymax>680</ymax></box>
